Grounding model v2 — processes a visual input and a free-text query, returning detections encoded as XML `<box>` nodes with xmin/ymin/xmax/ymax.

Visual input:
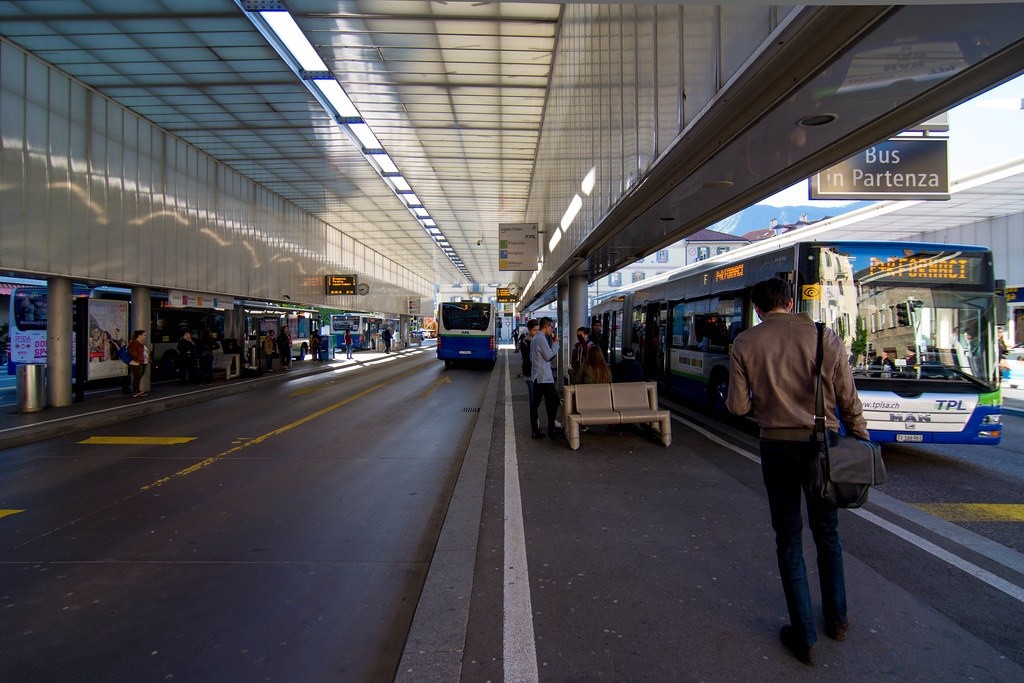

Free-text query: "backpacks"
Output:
<box><xmin>117</xmin><ymin>345</ymin><xmax>132</xmax><ymax>363</ymax></box>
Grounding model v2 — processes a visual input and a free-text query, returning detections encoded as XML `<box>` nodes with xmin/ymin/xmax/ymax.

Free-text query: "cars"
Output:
<box><xmin>412</xmin><ymin>330</ymin><xmax>437</xmax><ymax>341</ymax></box>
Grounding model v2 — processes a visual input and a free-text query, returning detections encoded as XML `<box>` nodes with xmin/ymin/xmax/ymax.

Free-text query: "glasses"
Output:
<box><xmin>545</xmin><ymin>324</ymin><xmax>554</xmax><ymax>329</ymax></box>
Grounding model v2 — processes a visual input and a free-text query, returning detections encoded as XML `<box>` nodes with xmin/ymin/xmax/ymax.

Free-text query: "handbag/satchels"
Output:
<box><xmin>184</xmin><ymin>348</ymin><xmax>193</xmax><ymax>360</ymax></box>
<box><xmin>810</xmin><ymin>427</ymin><xmax>888</xmax><ymax>507</ymax></box>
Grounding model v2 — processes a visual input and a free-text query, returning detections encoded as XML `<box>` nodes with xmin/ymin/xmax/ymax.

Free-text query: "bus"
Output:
<box><xmin>436</xmin><ymin>299</ymin><xmax>502</xmax><ymax>365</ymax></box>
<box><xmin>329</xmin><ymin>313</ymin><xmax>387</xmax><ymax>350</ymax></box>
<box><xmin>589</xmin><ymin>239</ymin><xmax>1007</xmax><ymax>447</ymax></box>
<box><xmin>7</xmin><ymin>284</ymin><xmax>249</xmax><ymax>381</ymax></box>
<box><xmin>243</xmin><ymin>311</ymin><xmax>325</xmax><ymax>362</ymax></box>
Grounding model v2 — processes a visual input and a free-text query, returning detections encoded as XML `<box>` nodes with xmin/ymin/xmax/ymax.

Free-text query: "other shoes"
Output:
<box><xmin>132</xmin><ymin>390</ymin><xmax>148</xmax><ymax>397</ymax></box>
<box><xmin>513</xmin><ymin>350</ymin><xmax>518</xmax><ymax>353</ymax></box>
<box><xmin>616</xmin><ymin>430</ymin><xmax>622</xmax><ymax>438</ymax></box>
<box><xmin>283</xmin><ymin>366</ymin><xmax>291</xmax><ymax>370</ymax></box>
<box><xmin>384</xmin><ymin>350</ymin><xmax>389</xmax><ymax>354</ymax></box>
<box><xmin>530</xmin><ymin>417</ymin><xmax>539</xmax><ymax>430</ymax></box>
<box><xmin>548</xmin><ymin>420</ymin><xmax>565</xmax><ymax>440</ymax></box>
<box><xmin>313</xmin><ymin>360</ymin><xmax>318</xmax><ymax>364</ymax></box>
<box><xmin>631</xmin><ymin>423</ymin><xmax>640</xmax><ymax>433</ymax></box>
<box><xmin>532</xmin><ymin>432</ymin><xmax>546</xmax><ymax>439</ymax></box>
<box><xmin>581</xmin><ymin>425</ymin><xmax>593</xmax><ymax>431</ymax></box>
<box><xmin>267</xmin><ymin>369</ymin><xmax>274</xmax><ymax>371</ymax></box>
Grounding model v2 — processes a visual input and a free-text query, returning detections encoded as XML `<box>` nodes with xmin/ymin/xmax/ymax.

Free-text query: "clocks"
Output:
<box><xmin>357</xmin><ymin>283</ymin><xmax>369</xmax><ymax>295</ymax></box>
<box><xmin>507</xmin><ymin>281</ymin><xmax>520</xmax><ymax>296</ymax></box>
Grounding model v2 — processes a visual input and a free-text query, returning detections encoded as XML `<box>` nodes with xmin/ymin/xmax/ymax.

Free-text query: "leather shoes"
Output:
<box><xmin>824</xmin><ymin>621</ymin><xmax>849</xmax><ymax>640</ymax></box>
<box><xmin>779</xmin><ymin>624</ymin><xmax>816</xmax><ymax>666</ymax></box>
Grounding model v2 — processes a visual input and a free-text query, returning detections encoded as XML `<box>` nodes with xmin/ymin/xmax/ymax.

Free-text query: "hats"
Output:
<box><xmin>91</xmin><ymin>328</ymin><xmax>100</xmax><ymax>336</ymax></box>
<box><xmin>622</xmin><ymin>348</ymin><xmax>635</xmax><ymax>360</ymax></box>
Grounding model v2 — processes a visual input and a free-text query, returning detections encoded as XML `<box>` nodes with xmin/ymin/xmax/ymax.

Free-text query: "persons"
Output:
<box><xmin>520</xmin><ymin>319</ymin><xmax>541</xmax><ymax>389</ymax></box>
<box><xmin>262</xmin><ymin>326</ymin><xmax>292</xmax><ymax>371</ymax></box>
<box><xmin>383</xmin><ymin>327</ymin><xmax>392</xmax><ymax>354</ymax></box>
<box><xmin>571</xmin><ymin>327</ymin><xmax>596</xmax><ymax>370</ymax></box>
<box><xmin>529</xmin><ymin>317</ymin><xmax>565</xmax><ymax>440</ymax></box>
<box><xmin>89</xmin><ymin>327</ymin><xmax>125</xmax><ymax>361</ymax></box>
<box><xmin>510</xmin><ymin>329</ymin><xmax>529</xmax><ymax>353</ymax></box>
<box><xmin>574</xmin><ymin>346</ymin><xmax>612</xmax><ymax>432</ymax></box>
<box><xmin>128</xmin><ymin>330</ymin><xmax>150</xmax><ymax>397</ymax></box>
<box><xmin>590</xmin><ymin>321</ymin><xmax>609</xmax><ymax>362</ymax></box>
<box><xmin>309</xmin><ymin>329</ymin><xmax>323</xmax><ymax>364</ymax></box>
<box><xmin>952</xmin><ymin>327</ymin><xmax>981</xmax><ymax>373</ymax></box>
<box><xmin>393</xmin><ymin>329</ymin><xmax>401</xmax><ymax>351</ymax></box>
<box><xmin>726</xmin><ymin>277</ymin><xmax>873</xmax><ymax>665</ymax></box>
<box><xmin>998</xmin><ymin>327</ymin><xmax>1011</xmax><ymax>384</ymax></box>
<box><xmin>611</xmin><ymin>348</ymin><xmax>647</xmax><ymax>430</ymax></box>
<box><xmin>344</xmin><ymin>329</ymin><xmax>353</xmax><ymax>359</ymax></box>
<box><xmin>176</xmin><ymin>330</ymin><xmax>220</xmax><ymax>386</ymax></box>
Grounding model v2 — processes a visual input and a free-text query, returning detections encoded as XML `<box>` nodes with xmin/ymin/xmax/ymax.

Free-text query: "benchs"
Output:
<box><xmin>212</xmin><ymin>354</ymin><xmax>240</xmax><ymax>380</ymax></box>
<box><xmin>561</xmin><ymin>381</ymin><xmax>673</xmax><ymax>450</ymax></box>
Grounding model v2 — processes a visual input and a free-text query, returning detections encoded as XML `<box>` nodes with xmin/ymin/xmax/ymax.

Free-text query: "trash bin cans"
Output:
<box><xmin>248</xmin><ymin>347</ymin><xmax>255</xmax><ymax>366</ymax></box>
<box><xmin>131</xmin><ymin>363</ymin><xmax>150</xmax><ymax>391</ymax></box>
<box><xmin>16</xmin><ymin>364</ymin><xmax>45</xmax><ymax>413</ymax></box>
<box><xmin>390</xmin><ymin>339</ymin><xmax>395</xmax><ymax>351</ymax></box>
